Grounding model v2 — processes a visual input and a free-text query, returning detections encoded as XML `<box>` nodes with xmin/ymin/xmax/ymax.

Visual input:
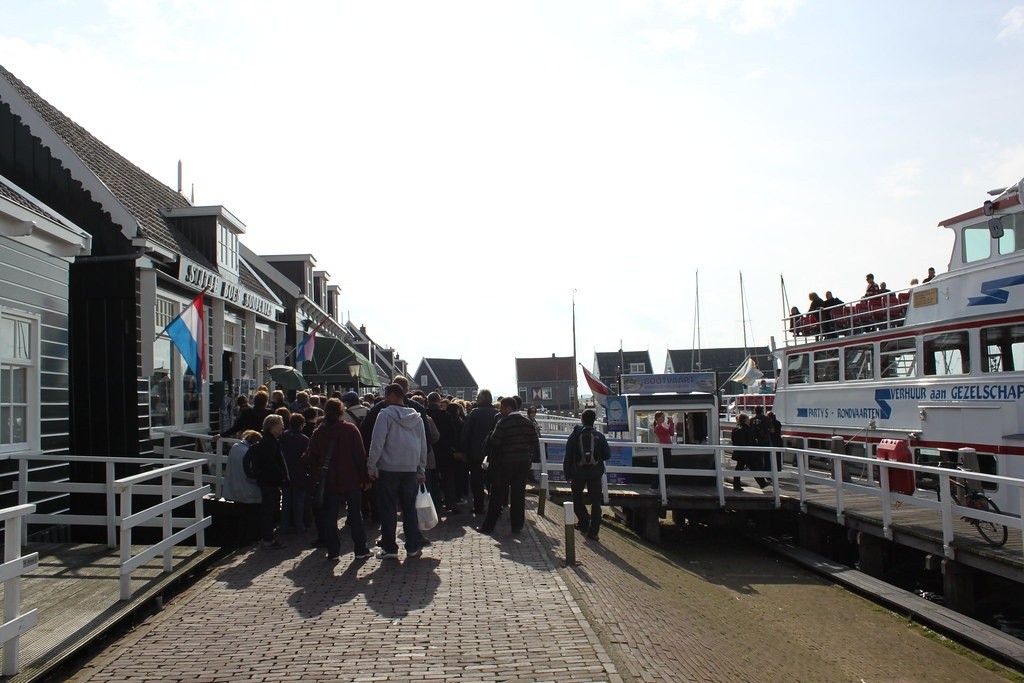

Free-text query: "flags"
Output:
<box><xmin>730</xmin><ymin>357</ymin><xmax>764</xmax><ymax>388</ymax></box>
<box><xmin>583</xmin><ymin>365</ymin><xmax>617</xmax><ymax>408</ymax></box>
<box><xmin>296</xmin><ymin>319</ymin><xmax>326</xmax><ymax>362</ymax></box>
<box><xmin>165</xmin><ymin>292</ymin><xmax>208</xmax><ymax>384</ymax></box>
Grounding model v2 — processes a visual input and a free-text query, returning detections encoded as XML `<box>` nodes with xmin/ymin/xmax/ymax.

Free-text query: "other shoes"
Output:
<box><xmin>648</xmin><ymin>488</ymin><xmax>656</xmax><ymax>493</ymax></box>
<box><xmin>446</xmin><ymin>504</ymin><xmax>462</xmax><ymax>514</ymax></box>
<box><xmin>263</xmin><ymin>540</ymin><xmax>287</xmax><ymax>549</ymax></box>
<box><xmin>416</xmin><ymin>536</ymin><xmax>430</xmax><ymax>545</ymax></box>
<box><xmin>760</xmin><ymin>481</ymin><xmax>772</xmax><ymax>489</ymax></box>
<box><xmin>470</xmin><ymin>508</ymin><xmax>484</xmax><ymax>514</ymax></box>
<box><xmin>330</xmin><ymin>556</ymin><xmax>340</xmax><ymax>560</ymax></box>
<box><xmin>355</xmin><ymin>552</ymin><xmax>373</xmax><ymax>559</ymax></box>
<box><xmin>588</xmin><ymin>533</ymin><xmax>598</xmax><ymax>540</ymax></box>
<box><xmin>512</xmin><ymin>529</ymin><xmax>520</xmax><ymax>532</ymax></box>
<box><xmin>476</xmin><ymin>526</ymin><xmax>492</xmax><ymax>532</ymax></box>
<box><xmin>375</xmin><ymin>539</ymin><xmax>383</xmax><ymax>547</ymax></box>
<box><xmin>574</xmin><ymin>523</ymin><xmax>586</xmax><ymax>531</ymax></box>
<box><xmin>734</xmin><ymin>487</ymin><xmax>743</xmax><ymax>491</ymax></box>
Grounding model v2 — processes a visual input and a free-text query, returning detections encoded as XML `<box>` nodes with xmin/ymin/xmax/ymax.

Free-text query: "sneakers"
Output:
<box><xmin>407</xmin><ymin>551</ymin><xmax>417</xmax><ymax>556</ymax></box>
<box><xmin>376</xmin><ymin>550</ymin><xmax>397</xmax><ymax>558</ymax></box>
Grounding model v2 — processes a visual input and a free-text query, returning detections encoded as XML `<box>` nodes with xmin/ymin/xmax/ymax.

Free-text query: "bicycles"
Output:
<box><xmin>915</xmin><ymin>449</ymin><xmax>1008</xmax><ymax>547</ymax></box>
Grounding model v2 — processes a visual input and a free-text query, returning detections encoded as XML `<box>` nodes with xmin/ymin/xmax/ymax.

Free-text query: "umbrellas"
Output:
<box><xmin>268</xmin><ymin>364</ymin><xmax>309</xmax><ymax>390</ymax></box>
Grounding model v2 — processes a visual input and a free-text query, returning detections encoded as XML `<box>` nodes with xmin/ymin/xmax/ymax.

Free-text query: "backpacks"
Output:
<box><xmin>572</xmin><ymin>428</ymin><xmax>600</xmax><ymax>468</ymax></box>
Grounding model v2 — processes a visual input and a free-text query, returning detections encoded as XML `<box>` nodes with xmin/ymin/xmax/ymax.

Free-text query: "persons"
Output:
<box><xmin>649</xmin><ymin>411</ymin><xmax>674</xmax><ymax>496</ymax></box>
<box><xmin>367</xmin><ymin>383</ymin><xmax>428</xmax><ymax>560</ymax></box>
<box><xmin>790</xmin><ymin>267</ymin><xmax>935</xmax><ymax>339</ymax></box>
<box><xmin>212</xmin><ymin>369</ymin><xmax>786</xmax><ymax>560</ymax></box>
<box><xmin>563</xmin><ymin>409</ymin><xmax>610</xmax><ymax>541</ymax></box>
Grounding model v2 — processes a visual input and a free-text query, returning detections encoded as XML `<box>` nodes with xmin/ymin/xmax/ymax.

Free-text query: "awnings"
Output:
<box><xmin>302</xmin><ymin>336</ymin><xmax>382</xmax><ymax>388</ymax></box>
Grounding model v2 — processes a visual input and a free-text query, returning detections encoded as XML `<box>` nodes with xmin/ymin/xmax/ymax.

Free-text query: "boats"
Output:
<box><xmin>720</xmin><ymin>176</ymin><xmax>1024</xmax><ymax>518</ymax></box>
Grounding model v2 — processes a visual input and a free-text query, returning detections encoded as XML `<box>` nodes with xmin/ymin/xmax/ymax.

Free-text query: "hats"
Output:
<box><xmin>342</xmin><ymin>391</ymin><xmax>358</xmax><ymax>400</ymax></box>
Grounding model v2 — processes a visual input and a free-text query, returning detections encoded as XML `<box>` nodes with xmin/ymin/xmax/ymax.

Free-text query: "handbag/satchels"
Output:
<box><xmin>310</xmin><ymin>470</ymin><xmax>327</xmax><ymax>506</ymax></box>
<box><xmin>415</xmin><ymin>482</ymin><xmax>439</xmax><ymax>530</ymax></box>
